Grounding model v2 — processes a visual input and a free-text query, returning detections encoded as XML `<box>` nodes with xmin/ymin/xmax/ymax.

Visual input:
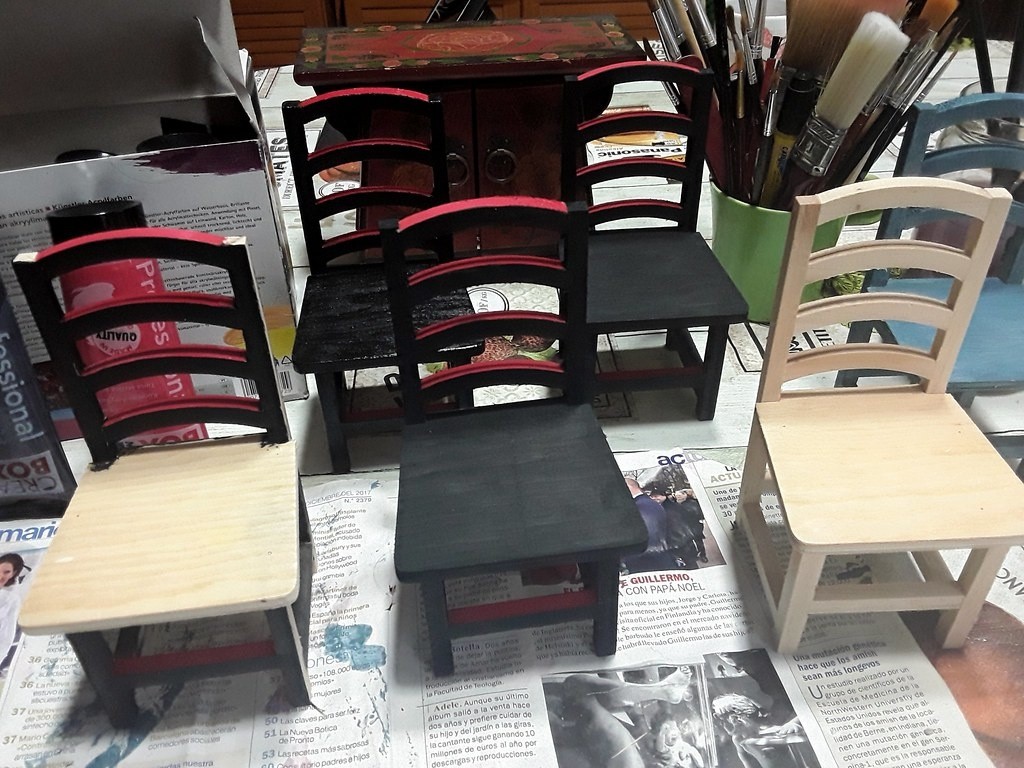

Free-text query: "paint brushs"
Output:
<box><xmin>631</xmin><ymin>0</ymin><xmax>976</xmax><ymax>212</ymax></box>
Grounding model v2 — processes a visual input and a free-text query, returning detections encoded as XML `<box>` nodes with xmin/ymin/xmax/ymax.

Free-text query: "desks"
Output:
<box><xmin>294</xmin><ymin>14</ymin><xmax>647</xmax><ymax>263</ymax></box>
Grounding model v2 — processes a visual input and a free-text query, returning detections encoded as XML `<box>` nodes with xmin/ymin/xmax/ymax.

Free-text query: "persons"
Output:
<box><xmin>545</xmin><ymin>667</ymin><xmax>821</xmax><ymax>768</ymax></box>
<box><xmin>619</xmin><ymin>479</ymin><xmax>708</xmax><ymax>571</ymax></box>
<box><xmin>0</xmin><ymin>553</ymin><xmax>25</xmax><ymax>668</ymax></box>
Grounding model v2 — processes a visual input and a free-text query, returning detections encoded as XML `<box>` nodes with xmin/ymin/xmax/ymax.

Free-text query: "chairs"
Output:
<box><xmin>834</xmin><ymin>93</ymin><xmax>1024</xmax><ymax>480</ymax></box>
<box><xmin>563</xmin><ymin>61</ymin><xmax>750</xmax><ymax>422</ymax></box>
<box><xmin>13</xmin><ymin>228</ymin><xmax>311</xmax><ymax>727</ymax></box>
<box><xmin>281</xmin><ymin>87</ymin><xmax>485</xmax><ymax>474</ymax></box>
<box><xmin>737</xmin><ymin>177</ymin><xmax>1024</xmax><ymax>655</ymax></box>
<box><xmin>378</xmin><ymin>199</ymin><xmax>648</xmax><ymax>674</ymax></box>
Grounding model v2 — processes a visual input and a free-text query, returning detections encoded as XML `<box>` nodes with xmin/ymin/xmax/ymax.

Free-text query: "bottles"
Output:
<box><xmin>38</xmin><ymin>199</ymin><xmax>204</xmax><ymax>453</ymax></box>
<box><xmin>901</xmin><ymin>78</ymin><xmax>1024</xmax><ymax>299</ymax></box>
<box><xmin>699</xmin><ymin>178</ymin><xmax>861</xmax><ymax>326</ymax></box>
